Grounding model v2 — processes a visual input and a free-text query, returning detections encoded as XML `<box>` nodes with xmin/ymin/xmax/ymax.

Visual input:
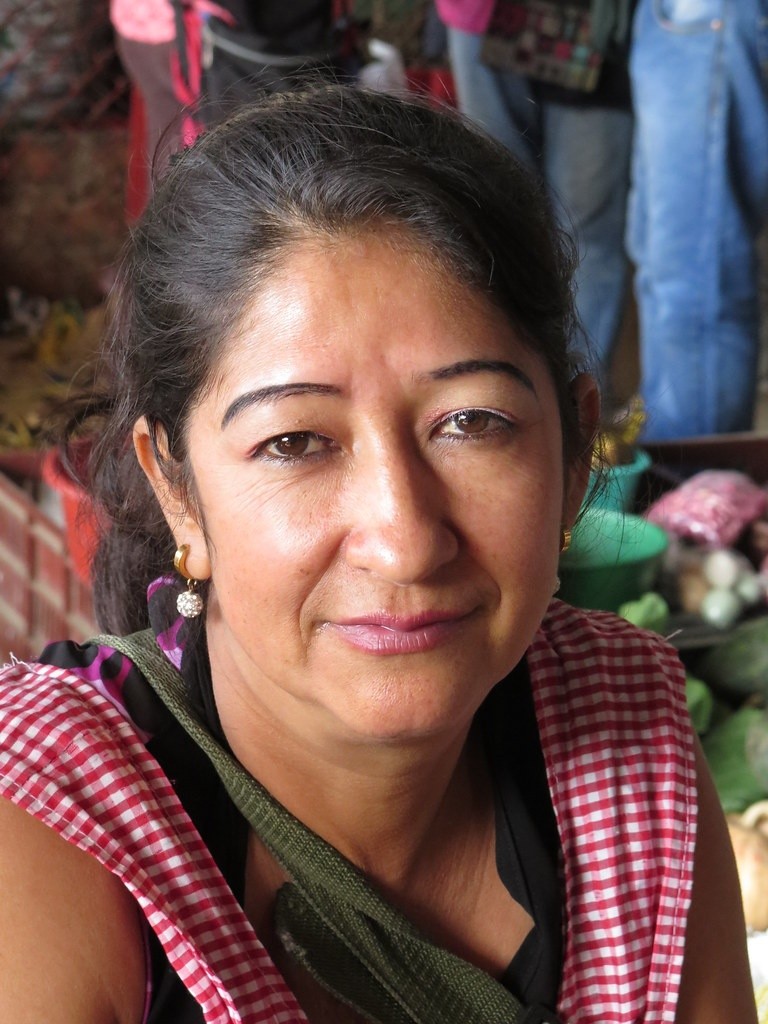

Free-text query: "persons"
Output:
<box><xmin>108</xmin><ymin>0</ymin><xmax>767</xmax><ymax>439</ymax></box>
<box><xmin>1</xmin><ymin>82</ymin><xmax>757</xmax><ymax>1024</ymax></box>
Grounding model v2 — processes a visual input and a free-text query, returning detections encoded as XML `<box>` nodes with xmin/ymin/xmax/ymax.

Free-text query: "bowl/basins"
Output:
<box><xmin>552</xmin><ymin>507</ymin><xmax>669</xmax><ymax>613</ymax></box>
<box><xmin>39</xmin><ymin>439</ymin><xmax>112</xmax><ymax>587</ymax></box>
<box><xmin>584</xmin><ymin>449</ymin><xmax>650</xmax><ymax>513</ymax></box>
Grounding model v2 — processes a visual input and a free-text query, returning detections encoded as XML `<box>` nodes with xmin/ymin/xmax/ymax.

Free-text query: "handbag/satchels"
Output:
<box><xmin>480</xmin><ymin>0</ymin><xmax>623</xmax><ymax>98</ymax></box>
